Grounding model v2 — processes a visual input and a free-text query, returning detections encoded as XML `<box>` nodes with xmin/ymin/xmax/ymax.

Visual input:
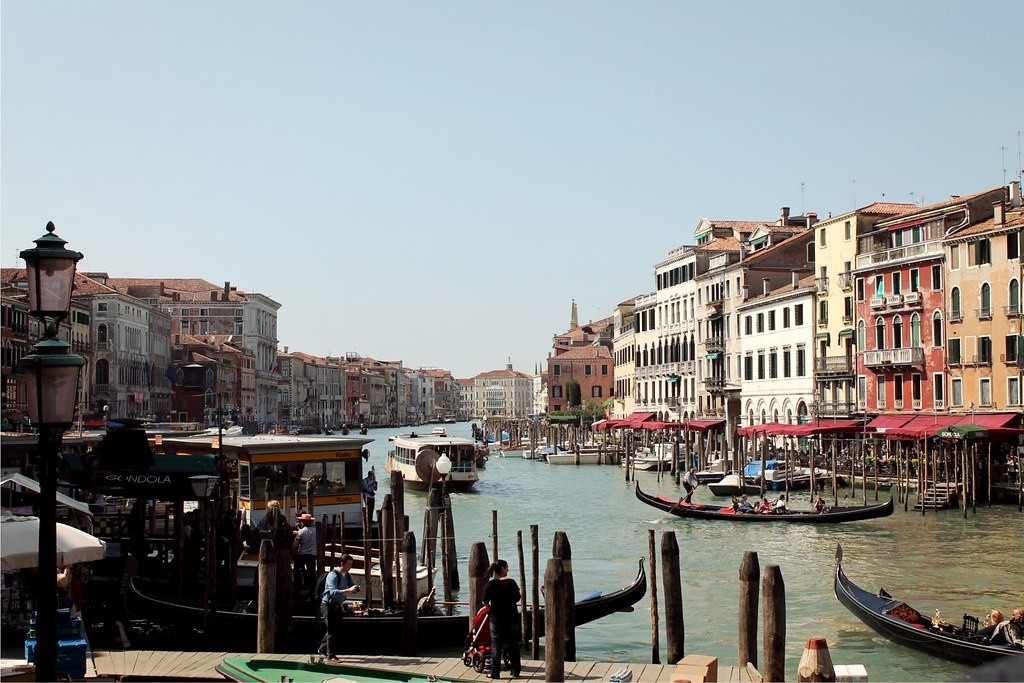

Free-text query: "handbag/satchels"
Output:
<box><xmin>369</xmin><ymin>491</ymin><xmax>375</xmax><ymax>496</ymax></box>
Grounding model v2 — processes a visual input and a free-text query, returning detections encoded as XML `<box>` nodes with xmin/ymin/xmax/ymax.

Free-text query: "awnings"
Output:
<box><xmin>860</xmin><ymin>414</ymin><xmax>1016</xmax><ymax>434</ymax></box>
<box><xmin>625</xmin><ymin>413</ymin><xmax>654</xmax><ymax>421</ymax></box>
<box><xmin>691</xmin><ymin>420</ymin><xmax>725</xmax><ymax>431</ymax></box>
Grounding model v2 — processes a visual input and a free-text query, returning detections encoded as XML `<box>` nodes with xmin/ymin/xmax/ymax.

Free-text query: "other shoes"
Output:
<box><xmin>330</xmin><ymin>656</ymin><xmax>342</xmax><ymax>663</ymax></box>
<box><xmin>487</xmin><ymin>674</ymin><xmax>499</xmax><ymax>679</ymax></box>
<box><xmin>318</xmin><ymin>649</ymin><xmax>327</xmax><ymax>656</ymax></box>
<box><xmin>510</xmin><ymin>672</ymin><xmax>520</xmax><ymax>679</ymax></box>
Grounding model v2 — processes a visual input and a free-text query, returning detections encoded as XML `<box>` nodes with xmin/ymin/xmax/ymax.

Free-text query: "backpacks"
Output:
<box><xmin>315</xmin><ymin>570</ymin><xmax>350</xmax><ymax>601</ymax></box>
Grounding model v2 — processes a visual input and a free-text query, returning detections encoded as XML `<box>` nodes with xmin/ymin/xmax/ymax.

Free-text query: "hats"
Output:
<box><xmin>368</xmin><ymin>471</ymin><xmax>374</xmax><ymax>475</ymax></box>
<box><xmin>267</xmin><ymin>500</ymin><xmax>280</xmax><ymax>511</ymax></box>
<box><xmin>296</xmin><ymin>513</ymin><xmax>316</xmax><ymax>520</ymax></box>
<box><xmin>295</xmin><ymin>510</ymin><xmax>307</xmax><ymax>517</ymax></box>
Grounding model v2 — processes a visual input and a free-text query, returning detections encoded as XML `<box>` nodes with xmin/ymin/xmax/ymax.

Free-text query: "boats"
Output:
<box><xmin>695</xmin><ymin>456</ymin><xmax>843</xmax><ymax>497</ymax></box>
<box><xmin>1</xmin><ymin>425</ymin><xmax>438</xmax><ymax>605</ymax></box>
<box><xmin>444</xmin><ymin>414</ymin><xmax>456</xmax><ymax>423</ymax></box>
<box><xmin>834</xmin><ymin>543</ymin><xmax>1024</xmax><ymax>666</ymax></box>
<box><xmin>635</xmin><ymin>480</ymin><xmax>895</xmax><ymax>523</ymax></box>
<box><xmin>127</xmin><ymin>556</ymin><xmax>648</xmax><ymax>656</ymax></box>
<box><xmin>620</xmin><ymin>457</ymin><xmax>671</xmax><ymax>472</ymax></box>
<box><xmin>214</xmin><ymin>656</ymin><xmax>485</xmax><ymax>682</ymax></box>
<box><xmin>478</xmin><ymin>431</ymin><xmax>623</xmax><ymax>465</ymax></box>
<box><xmin>385</xmin><ymin>426</ymin><xmax>477</xmax><ymax>489</ymax></box>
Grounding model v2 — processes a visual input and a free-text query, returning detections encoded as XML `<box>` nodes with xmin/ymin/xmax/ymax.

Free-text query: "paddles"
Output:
<box><xmin>668</xmin><ymin>484</ymin><xmax>700</xmax><ymax>513</ymax></box>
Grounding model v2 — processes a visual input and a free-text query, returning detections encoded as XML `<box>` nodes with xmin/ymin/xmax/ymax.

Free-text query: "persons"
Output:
<box><xmin>257</xmin><ymin>500</ymin><xmax>317</xmax><ymax>599</ymax></box>
<box><xmin>16</xmin><ymin>570</ymin><xmax>77</xmax><ymax>618</ymax></box>
<box><xmin>363</xmin><ymin>471</ymin><xmax>378</xmax><ymax>523</ymax></box>
<box><xmin>978</xmin><ymin>606</ymin><xmax>1024</xmax><ymax>649</ymax></box>
<box><xmin>450</xmin><ymin>454</ymin><xmax>455</xmax><ymax>471</ymax></box>
<box><xmin>314</xmin><ymin>473</ymin><xmax>342</xmax><ymax>488</ymax></box>
<box><xmin>814</xmin><ymin>495</ymin><xmax>825</xmax><ymax>511</ymax></box>
<box><xmin>1006</xmin><ymin>454</ymin><xmax>1016</xmax><ymax>480</ymax></box>
<box><xmin>683</xmin><ymin>468</ymin><xmax>704</xmax><ymax>504</ymax></box>
<box><xmin>474</xmin><ymin>431</ymin><xmax>489</xmax><ymax>451</ymax></box>
<box><xmin>482</xmin><ymin>560</ymin><xmax>521</xmax><ymax>679</ymax></box>
<box><xmin>179</xmin><ymin>504</ymin><xmax>236</xmax><ymax>586</ymax></box>
<box><xmin>729</xmin><ymin>494</ymin><xmax>786</xmax><ymax>514</ymax></box>
<box><xmin>317</xmin><ymin>554</ymin><xmax>360</xmax><ymax>663</ymax></box>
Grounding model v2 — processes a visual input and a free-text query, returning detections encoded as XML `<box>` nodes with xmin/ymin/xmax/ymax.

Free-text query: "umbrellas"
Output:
<box><xmin>1</xmin><ymin>510</ymin><xmax>107</xmax><ymax>573</ymax></box>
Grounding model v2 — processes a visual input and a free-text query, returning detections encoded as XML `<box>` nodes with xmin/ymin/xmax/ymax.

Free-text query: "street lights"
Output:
<box><xmin>17</xmin><ymin>220</ymin><xmax>88</xmax><ymax>682</ymax></box>
